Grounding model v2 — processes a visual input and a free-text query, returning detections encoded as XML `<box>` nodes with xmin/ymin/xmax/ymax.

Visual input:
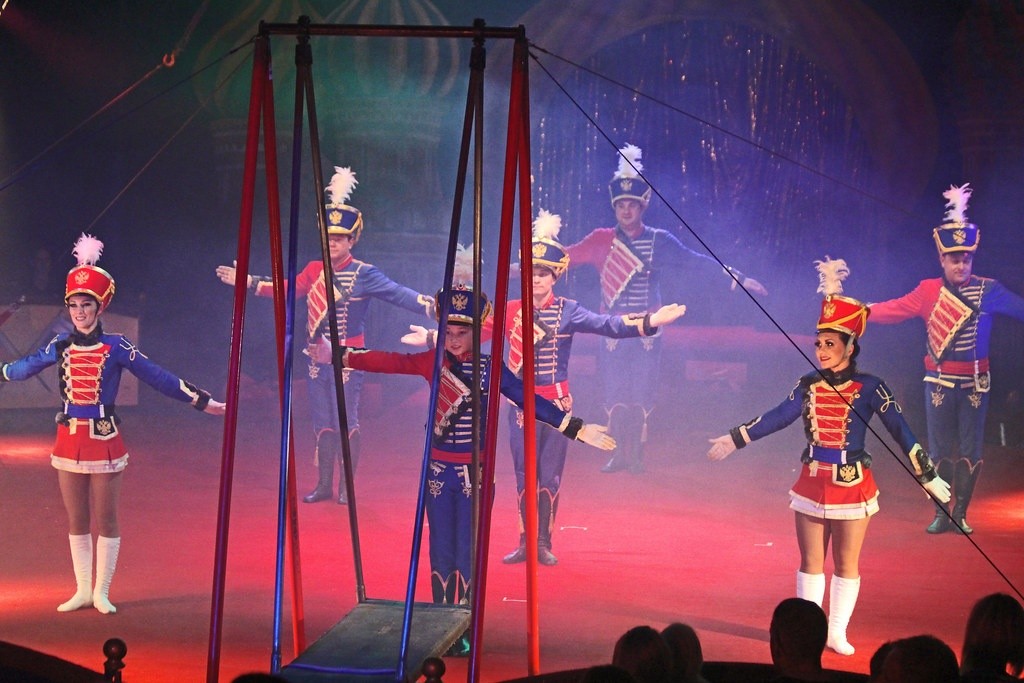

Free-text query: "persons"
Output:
<box><xmin>0</xmin><ymin>233</ymin><xmax>228</xmax><ymax>614</ymax></box>
<box><xmin>217</xmin><ymin>165</ymin><xmax>440</xmax><ymax>503</ymax></box>
<box><xmin>596</xmin><ymin>592</ymin><xmax>1023</xmax><ymax>683</ymax></box>
<box><xmin>304</xmin><ymin>144</ymin><xmax>770</xmax><ymax>567</ymax></box>
<box><xmin>709</xmin><ymin>259</ymin><xmax>951</xmax><ymax>656</ymax></box>
<box><xmin>863</xmin><ymin>184</ymin><xmax>1024</xmax><ymax>537</ymax></box>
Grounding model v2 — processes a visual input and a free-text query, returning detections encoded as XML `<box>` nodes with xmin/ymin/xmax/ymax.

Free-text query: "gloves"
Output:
<box><xmin>650</xmin><ymin>303</ymin><xmax>686</xmax><ymax>326</ymax></box>
<box><xmin>743</xmin><ymin>278</ymin><xmax>768</xmax><ymax>297</ymax></box>
<box><xmin>216</xmin><ymin>261</ymin><xmax>251</xmax><ymax>287</ymax></box>
<box><xmin>509</xmin><ymin>263</ymin><xmax>521</xmax><ymax>279</ymax></box>
<box><xmin>205</xmin><ymin>396</ymin><xmax>227</xmax><ymax>416</ymax></box>
<box><xmin>578</xmin><ymin>424</ymin><xmax>617</xmax><ymax>452</ymax></box>
<box><xmin>308</xmin><ymin>335</ymin><xmax>332</xmax><ymax>365</ymax></box>
<box><xmin>922</xmin><ymin>476</ymin><xmax>951</xmax><ymax>503</ymax></box>
<box><xmin>400</xmin><ymin>325</ymin><xmax>429</xmax><ymax>345</ymax></box>
<box><xmin>706</xmin><ymin>434</ymin><xmax>737</xmax><ymax>462</ymax></box>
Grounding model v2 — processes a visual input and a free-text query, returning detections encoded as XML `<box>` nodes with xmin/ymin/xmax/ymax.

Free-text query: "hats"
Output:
<box><xmin>813</xmin><ymin>256</ymin><xmax>871</xmax><ymax>338</ymax></box>
<box><xmin>518</xmin><ymin>210</ymin><xmax>571</xmax><ymax>280</ymax></box>
<box><xmin>434</xmin><ymin>241</ymin><xmax>493</xmax><ymax>327</ymax></box>
<box><xmin>609</xmin><ymin>143</ymin><xmax>651</xmax><ymax>208</ymax></box>
<box><xmin>934</xmin><ymin>183</ymin><xmax>981</xmax><ymax>254</ymax></box>
<box><xmin>316</xmin><ymin>168</ymin><xmax>364</xmax><ymax>242</ymax></box>
<box><xmin>65</xmin><ymin>233</ymin><xmax>115</xmax><ymax>308</ymax></box>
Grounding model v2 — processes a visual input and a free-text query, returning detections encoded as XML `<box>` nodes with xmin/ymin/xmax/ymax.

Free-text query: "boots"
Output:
<box><xmin>502</xmin><ymin>486</ymin><xmax>562</xmax><ymax>565</ymax></box>
<box><xmin>927</xmin><ymin>458</ymin><xmax>983</xmax><ymax>534</ymax></box>
<box><xmin>56</xmin><ymin>534</ymin><xmax>121</xmax><ymax>613</ymax></box>
<box><xmin>827</xmin><ymin>575</ymin><xmax>860</xmax><ymax>655</ymax></box>
<box><xmin>304</xmin><ymin>424</ymin><xmax>358</xmax><ymax>505</ymax></box>
<box><xmin>430</xmin><ymin>570</ymin><xmax>475</xmax><ymax>657</ymax></box>
<box><xmin>601</xmin><ymin>402</ymin><xmax>657</xmax><ymax>474</ymax></box>
<box><xmin>796</xmin><ymin>571</ymin><xmax>826</xmax><ymax>608</ymax></box>
